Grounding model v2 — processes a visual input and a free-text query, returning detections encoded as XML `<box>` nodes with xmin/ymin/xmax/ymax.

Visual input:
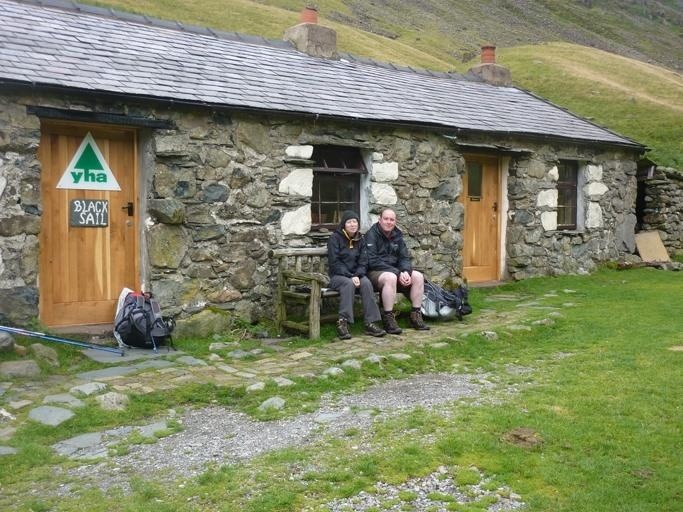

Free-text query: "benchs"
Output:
<box><xmin>269</xmin><ymin>244</ymin><xmax>427</xmax><ymax>341</ymax></box>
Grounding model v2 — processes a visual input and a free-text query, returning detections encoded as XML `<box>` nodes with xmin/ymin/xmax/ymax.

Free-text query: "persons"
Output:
<box><xmin>364</xmin><ymin>209</ymin><xmax>431</xmax><ymax>333</ymax></box>
<box><xmin>327</xmin><ymin>210</ymin><xmax>386</xmax><ymax>339</ymax></box>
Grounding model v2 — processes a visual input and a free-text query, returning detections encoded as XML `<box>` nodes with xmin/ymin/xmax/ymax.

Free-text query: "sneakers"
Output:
<box><xmin>335</xmin><ymin>320</ymin><xmax>351</xmax><ymax>340</ymax></box>
<box><xmin>360</xmin><ymin>323</ymin><xmax>386</xmax><ymax>337</ymax></box>
<box><xmin>409</xmin><ymin>309</ymin><xmax>429</xmax><ymax>330</ymax></box>
<box><xmin>382</xmin><ymin>313</ymin><xmax>401</xmax><ymax>334</ymax></box>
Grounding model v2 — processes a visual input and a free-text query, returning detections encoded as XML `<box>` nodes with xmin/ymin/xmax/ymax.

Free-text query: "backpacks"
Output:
<box><xmin>420</xmin><ymin>274</ymin><xmax>462</xmax><ymax>322</ymax></box>
<box><xmin>113</xmin><ymin>291</ymin><xmax>177</xmax><ymax>353</ymax></box>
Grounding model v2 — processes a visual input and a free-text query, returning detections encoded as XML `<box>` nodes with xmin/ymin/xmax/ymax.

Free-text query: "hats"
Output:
<box><xmin>340</xmin><ymin>210</ymin><xmax>359</xmax><ymax>229</ymax></box>
